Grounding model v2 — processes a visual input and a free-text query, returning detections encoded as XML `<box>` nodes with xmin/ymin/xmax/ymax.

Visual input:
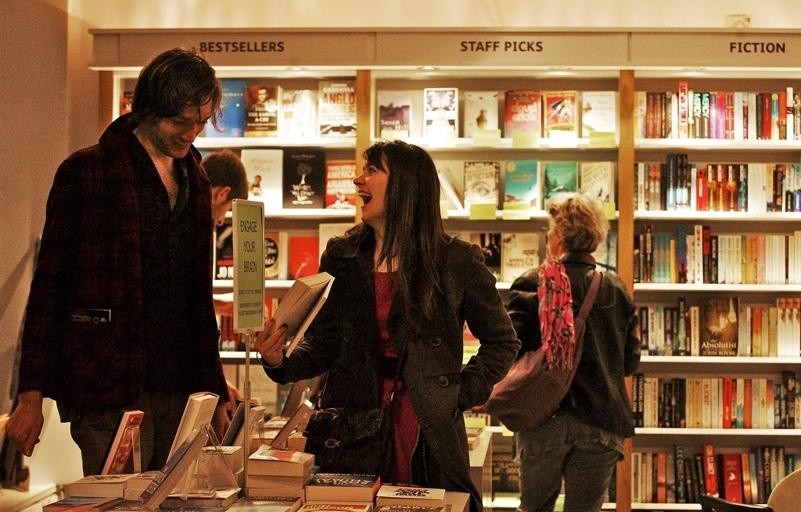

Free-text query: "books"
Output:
<box><xmin>118</xmin><ymin>77</ymin><xmax>358</xmax><ymax>350</ymax></box>
<box><xmin>379</xmin><ymin>88</ymin><xmax>623</xmax><ymax>287</ymax></box>
<box><xmin>39</xmin><ymin>325</ymin><xmax>618</xmax><ymax>512</ymax></box>
<box><xmin>626</xmin><ymin>70</ymin><xmax>801</xmax><ymax>510</ymax></box>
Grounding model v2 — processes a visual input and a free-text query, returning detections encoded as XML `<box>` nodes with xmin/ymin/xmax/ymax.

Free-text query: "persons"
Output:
<box><xmin>505</xmin><ymin>192</ymin><xmax>642</xmax><ymax>512</ymax></box>
<box><xmin>4</xmin><ymin>46</ymin><xmax>239</xmax><ymax>479</ymax></box>
<box><xmin>254</xmin><ymin>139</ymin><xmax>521</xmax><ymax>512</ymax></box>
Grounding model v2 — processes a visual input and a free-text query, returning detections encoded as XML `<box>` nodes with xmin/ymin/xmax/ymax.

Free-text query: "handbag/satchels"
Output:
<box><xmin>478</xmin><ymin>268</ymin><xmax>603</xmax><ymax>434</ymax></box>
<box><xmin>303</xmin><ymin>403</ymin><xmax>401</xmax><ymax>484</ymax></box>
